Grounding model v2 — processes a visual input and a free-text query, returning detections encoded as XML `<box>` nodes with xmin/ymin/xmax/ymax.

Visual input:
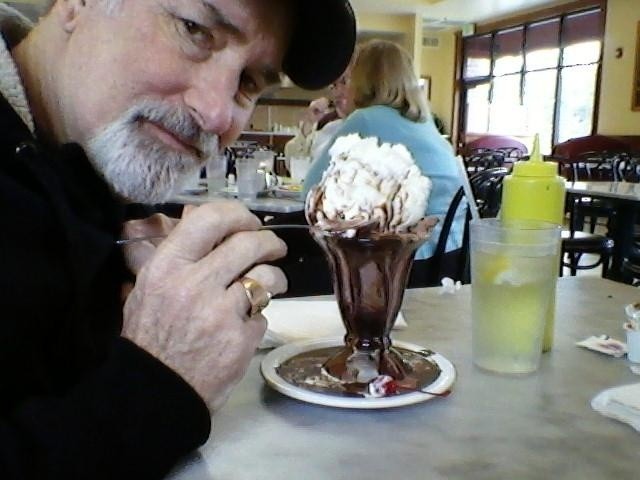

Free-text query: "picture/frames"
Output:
<box><xmin>421</xmin><ymin>75</ymin><xmax>432</xmax><ymax>100</ymax></box>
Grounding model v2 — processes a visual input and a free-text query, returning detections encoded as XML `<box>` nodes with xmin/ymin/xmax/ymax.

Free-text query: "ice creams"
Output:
<box><xmin>303</xmin><ymin>132</ymin><xmax>439</xmax><ymax>382</ymax></box>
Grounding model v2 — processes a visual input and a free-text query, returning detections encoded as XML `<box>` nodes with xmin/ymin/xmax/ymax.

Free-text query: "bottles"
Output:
<box><xmin>499</xmin><ymin>132</ymin><xmax>567</xmax><ymax>353</ymax></box>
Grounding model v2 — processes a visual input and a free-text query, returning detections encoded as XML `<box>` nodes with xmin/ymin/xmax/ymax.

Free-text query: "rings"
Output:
<box><xmin>237</xmin><ymin>278</ymin><xmax>270</xmax><ymax>320</ymax></box>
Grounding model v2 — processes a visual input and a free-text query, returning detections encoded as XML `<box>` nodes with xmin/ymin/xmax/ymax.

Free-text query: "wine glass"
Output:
<box><xmin>319</xmin><ymin>215</ymin><xmax>440</xmax><ymax>389</ymax></box>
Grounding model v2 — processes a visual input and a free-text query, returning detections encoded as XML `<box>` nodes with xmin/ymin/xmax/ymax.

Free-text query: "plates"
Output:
<box><xmin>182</xmin><ymin>185</ymin><xmax>205</xmax><ymax>195</ymax></box>
<box><xmin>272</xmin><ymin>184</ymin><xmax>306</xmax><ymax>198</ymax></box>
<box><xmin>260</xmin><ymin>335</ymin><xmax>457</xmax><ymax>410</ymax></box>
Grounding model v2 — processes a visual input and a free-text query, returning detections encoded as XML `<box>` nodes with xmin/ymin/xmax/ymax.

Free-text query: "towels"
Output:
<box><xmin>257</xmin><ymin>300</ymin><xmax>408</xmax><ymax>350</ymax></box>
<box><xmin>590</xmin><ymin>384</ymin><xmax>640</xmax><ymax>432</ymax></box>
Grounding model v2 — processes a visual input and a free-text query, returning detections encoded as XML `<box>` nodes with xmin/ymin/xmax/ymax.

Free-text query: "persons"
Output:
<box><xmin>1</xmin><ymin>1</ymin><xmax>356</xmax><ymax>480</ymax></box>
<box><xmin>302</xmin><ymin>38</ymin><xmax>468</xmax><ymax>289</ymax></box>
<box><xmin>284</xmin><ymin>55</ymin><xmax>355</xmax><ymax>177</ymax></box>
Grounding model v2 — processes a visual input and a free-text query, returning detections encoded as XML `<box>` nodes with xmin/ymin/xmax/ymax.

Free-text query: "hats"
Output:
<box><xmin>284</xmin><ymin>0</ymin><xmax>356</xmax><ymax>89</ymax></box>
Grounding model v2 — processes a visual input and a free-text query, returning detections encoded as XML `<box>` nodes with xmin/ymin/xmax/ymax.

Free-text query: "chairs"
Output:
<box><xmin>429</xmin><ymin>146</ymin><xmax>640</xmax><ymax>286</ymax></box>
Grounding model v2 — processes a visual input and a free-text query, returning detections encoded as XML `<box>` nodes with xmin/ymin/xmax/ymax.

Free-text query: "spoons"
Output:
<box><xmin>117</xmin><ymin>214</ymin><xmax>383</xmax><ymax>245</ymax></box>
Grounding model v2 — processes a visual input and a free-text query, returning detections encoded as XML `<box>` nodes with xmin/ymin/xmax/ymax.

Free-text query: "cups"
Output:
<box><xmin>199</xmin><ymin>148</ymin><xmax>281</xmax><ymax>204</ymax></box>
<box><xmin>469</xmin><ymin>218</ymin><xmax>564</xmax><ymax>376</ymax></box>
